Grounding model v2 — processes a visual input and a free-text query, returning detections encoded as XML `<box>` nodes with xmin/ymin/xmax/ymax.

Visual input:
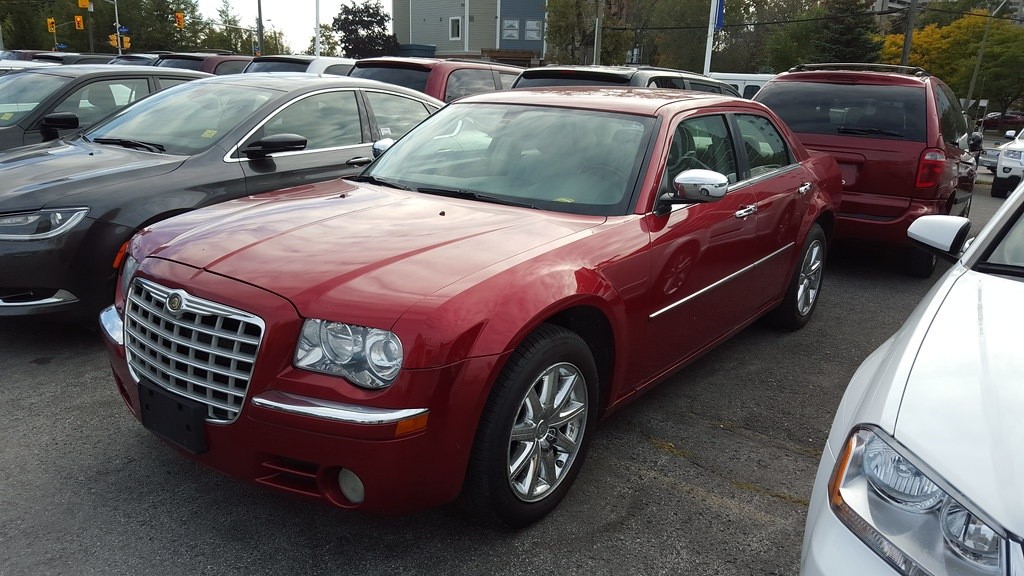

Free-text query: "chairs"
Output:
<box><xmin>87</xmin><ymin>83</ymin><xmax>117</xmax><ymax>106</ymax></box>
<box><xmin>510</xmin><ymin>118</ymin><xmax>577</xmax><ymax>182</ymax></box>
<box><xmin>666</xmin><ymin>126</ymin><xmax>709</xmax><ymax>192</ymax></box>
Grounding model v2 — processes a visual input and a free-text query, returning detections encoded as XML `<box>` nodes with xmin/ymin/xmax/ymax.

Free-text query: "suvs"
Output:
<box><xmin>1</xmin><ymin>47</ymin><xmax>985</xmax><ymax>280</ymax></box>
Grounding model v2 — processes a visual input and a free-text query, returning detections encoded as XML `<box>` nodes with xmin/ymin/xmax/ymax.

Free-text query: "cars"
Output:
<box><xmin>977</xmin><ymin>110</ymin><xmax>1024</xmax><ymax>132</ymax></box>
<box><xmin>0</xmin><ymin>69</ymin><xmax>495</xmax><ymax>332</ymax></box>
<box><xmin>800</xmin><ymin>179</ymin><xmax>1024</xmax><ymax>576</ymax></box>
<box><xmin>0</xmin><ymin>63</ymin><xmax>223</xmax><ymax>155</ymax></box>
<box><xmin>100</xmin><ymin>82</ymin><xmax>848</xmax><ymax>530</ymax></box>
<box><xmin>991</xmin><ymin>128</ymin><xmax>1024</xmax><ymax>198</ymax></box>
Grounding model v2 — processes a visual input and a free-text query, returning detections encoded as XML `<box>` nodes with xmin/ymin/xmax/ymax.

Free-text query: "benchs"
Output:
<box><xmin>603</xmin><ymin>125</ymin><xmax>759</xmax><ymax>177</ymax></box>
